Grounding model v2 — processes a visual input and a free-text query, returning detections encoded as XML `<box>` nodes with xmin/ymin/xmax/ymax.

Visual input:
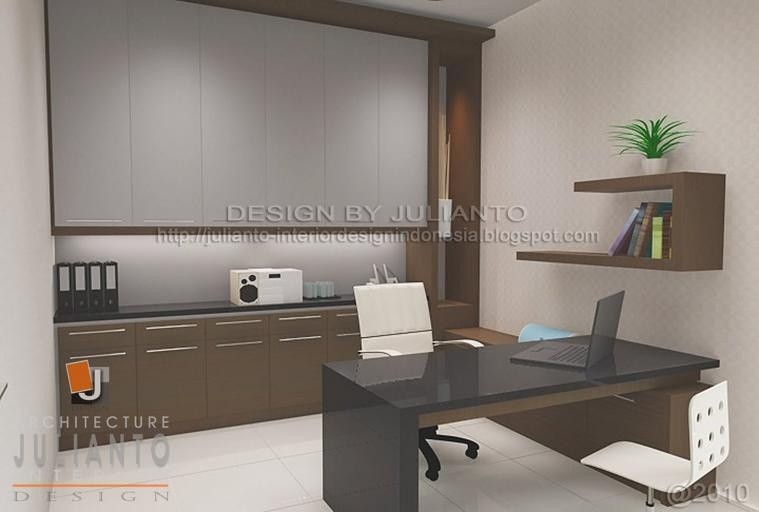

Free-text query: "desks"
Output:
<box><xmin>319</xmin><ymin>336</ymin><xmax>720</xmax><ymax>510</ymax></box>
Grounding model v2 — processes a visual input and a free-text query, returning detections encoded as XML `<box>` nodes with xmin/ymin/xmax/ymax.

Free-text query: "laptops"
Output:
<box><xmin>509</xmin><ymin>291</ymin><xmax>626</xmax><ymax>369</ymax></box>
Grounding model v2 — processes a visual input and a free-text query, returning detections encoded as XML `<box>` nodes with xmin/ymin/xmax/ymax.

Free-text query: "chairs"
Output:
<box><xmin>353</xmin><ymin>280</ymin><xmax>480</xmax><ymax>482</ymax></box>
<box><xmin>578</xmin><ymin>379</ymin><xmax>731</xmax><ymax>507</ymax></box>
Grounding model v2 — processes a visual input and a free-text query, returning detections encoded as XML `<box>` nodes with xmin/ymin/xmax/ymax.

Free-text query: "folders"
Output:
<box><xmin>57</xmin><ymin>261</ymin><xmax>119</xmax><ymax>314</ymax></box>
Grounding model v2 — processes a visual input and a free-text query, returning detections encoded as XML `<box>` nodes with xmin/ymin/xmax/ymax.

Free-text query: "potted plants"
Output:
<box><xmin>613</xmin><ymin>115</ymin><xmax>696</xmax><ymax>173</ymax></box>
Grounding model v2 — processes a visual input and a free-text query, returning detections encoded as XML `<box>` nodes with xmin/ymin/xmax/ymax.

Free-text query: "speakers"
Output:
<box><xmin>229</xmin><ymin>268</ymin><xmax>303</xmax><ymax>307</ymax></box>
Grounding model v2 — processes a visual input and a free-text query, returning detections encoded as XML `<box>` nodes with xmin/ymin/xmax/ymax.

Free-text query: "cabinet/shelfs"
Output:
<box><xmin>448</xmin><ymin>327</ymin><xmax>722</xmax><ymax>508</ymax></box>
<box><xmin>513</xmin><ymin>169</ymin><xmax>723</xmax><ymax>273</ymax></box>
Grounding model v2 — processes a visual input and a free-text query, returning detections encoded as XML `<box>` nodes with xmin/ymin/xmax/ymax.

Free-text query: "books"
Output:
<box><xmin>607</xmin><ymin>203</ymin><xmax>672</xmax><ymax>260</ymax></box>
